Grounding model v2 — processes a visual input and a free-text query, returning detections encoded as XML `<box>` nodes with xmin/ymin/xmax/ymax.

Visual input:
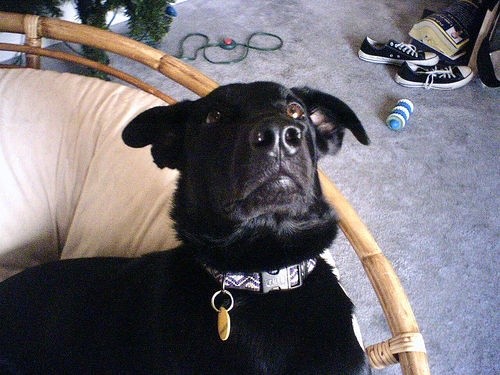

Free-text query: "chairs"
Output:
<box><xmin>0</xmin><ymin>11</ymin><xmax>431</xmax><ymax>374</ymax></box>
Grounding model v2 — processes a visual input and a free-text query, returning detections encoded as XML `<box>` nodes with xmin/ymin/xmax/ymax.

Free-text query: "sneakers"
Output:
<box><xmin>394</xmin><ymin>61</ymin><xmax>474</xmax><ymax>90</ymax></box>
<box><xmin>358</xmin><ymin>35</ymin><xmax>439</xmax><ymax>67</ymax></box>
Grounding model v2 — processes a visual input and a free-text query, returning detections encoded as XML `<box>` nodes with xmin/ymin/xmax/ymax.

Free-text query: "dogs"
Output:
<box><xmin>0</xmin><ymin>80</ymin><xmax>371</xmax><ymax>375</ymax></box>
